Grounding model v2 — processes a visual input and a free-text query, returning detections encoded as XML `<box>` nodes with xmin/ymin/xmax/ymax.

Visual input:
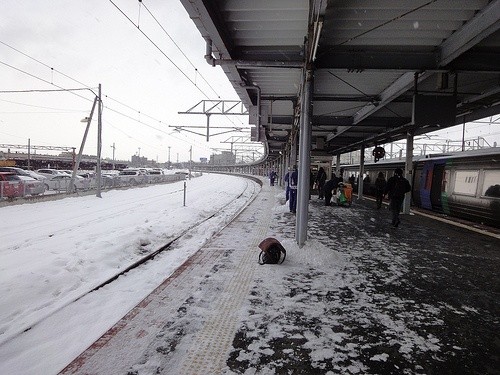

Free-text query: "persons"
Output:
<box><xmin>375</xmin><ymin>172</ymin><xmax>388</xmax><ymax>208</ymax></box>
<box><xmin>363</xmin><ymin>175</ymin><xmax>370</xmax><ymax>197</ymax></box>
<box><xmin>309</xmin><ymin>169</ymin><xmax>315</xmax><ymax>199</ymax></box>
<box><xmin>387</xmin><ymin>169</ymin><xmax>411</xmax><ymax>228</ymax></box>
<box><xmin>269</xmin><ymin>170</ymin><xmax>277</xmax><ymax>186</ymax></box>
<box><xmin>322</xmin><ymin>177</ymin><xmax>341</xmax><ymax>205</ymax></box>
<box><xmin>339</xmin><ymin>174</ymin><xmax>343</xmax><ymax>182</ymax></box>
<box><xmin>349</xmin><ymin>174</ymin><xmax>355</xmax><ymax>191</ymax></box>
<box><xmin>285</xmin><ymin>168</ymin><xmax>298</xmax><ymax>213</ymax></box>
<box><xmin>315</xmin><ymin>167</ymin><xmax>326</xmax><ymax>200</ymax></box>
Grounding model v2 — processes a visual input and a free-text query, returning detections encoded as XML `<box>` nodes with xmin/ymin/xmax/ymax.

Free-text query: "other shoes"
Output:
<box><xmin>317</xmin><ymin>197</ymin><xmax>323</xmax><ymax>200</ymax></box>
<box><xmin>325</xmin><ymin>203</ymin><xmax>331</xmax><ymax>206</ymax></box>
<box><xmin>377</xmin><ymin>203</ymin><xmax>381</xmax><ymax>210</ymax></box>
<box><xmin>290</xmin><ymin>210</ymin><xmax>296</xmax><ymax>213</ymax></box>
<box><xmin>392</xmin><ymin>220</ymin><xmax>400</xmax><ymax>227</ymax></box>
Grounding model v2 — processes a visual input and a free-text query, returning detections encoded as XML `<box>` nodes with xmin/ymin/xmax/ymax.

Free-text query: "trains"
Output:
<box><xmin>330</xmin><ymin>146</ymin><xmax>500</xmax><ymax>230</ymax></box>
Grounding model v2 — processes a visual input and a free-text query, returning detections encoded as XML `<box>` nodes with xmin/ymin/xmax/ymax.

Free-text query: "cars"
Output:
<box><xmin>0</xmin><ymin>167</ymin><xmax>47</xmax><ymax>185</ymax></box>
<box><xmin>34</xmin><ymin>167</ymin><xmax>165</xmax><ymax>185</ymax></box>
<box><xmin>17</xmin><ymin>175</ymin><xmax>44</xmax><ymax>197</ymax></box>
<box><xmin>0</xmin><ymin>171</ymin><xmax>26</xmax><ymax>199</ymax></box>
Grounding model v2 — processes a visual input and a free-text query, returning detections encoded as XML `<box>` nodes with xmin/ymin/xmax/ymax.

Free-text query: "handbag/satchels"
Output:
<box><xmin>317</xmin><ymin>178</ymin><xmax>322</xmax><ymax>186</ymax></box>
